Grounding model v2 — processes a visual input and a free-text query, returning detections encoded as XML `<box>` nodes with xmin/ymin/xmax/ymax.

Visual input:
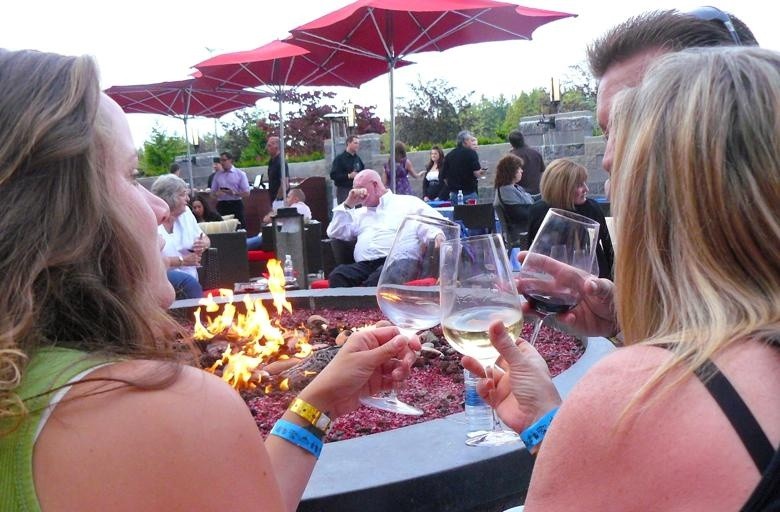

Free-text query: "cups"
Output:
<box><xmin>205</xmin><ymin>187</ymin><xmax>210</xmax><ymax>192</ymax></box>
<box><xmin>547</xmin><ymin>243</ymin><xmax>601</xmax><ymax>282</ymax></box>
<box><xmin>199</xmin><ymin>219</ymin><xmax>239</xmax><ymax>232</ymax></box>
<box><xmin>200</xmin><ymin>189</ymin><xmax>203</xmax><ymax>191</ymax></box>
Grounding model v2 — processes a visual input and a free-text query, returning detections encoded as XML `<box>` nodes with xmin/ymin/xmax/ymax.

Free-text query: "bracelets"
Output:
<box><xmin>519</xmin><ymin>406</ymin><xmax>564</xmax><ymax>449</ymax></box>
<box><xmin>609</xmin><ymin>330</ymin><xmax>625</xmax><ymax>346</ymax></box>
<box><xmin>269</xmin><ymin>418</ymin><xmax>324</xmax><ymax>457</ymax></box>
<box><xmin>288</xmin><ymin>398</ymin><xmax>336</xmax><ymax>437</ymax></box>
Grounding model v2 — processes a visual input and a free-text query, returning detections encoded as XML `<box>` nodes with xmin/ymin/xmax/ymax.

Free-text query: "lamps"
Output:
<box><xmin>346</xmin><ymin>99</ymin><xmax>356</xmax><ymax>135</ymax></box>
<box><xmin>537</xmin><ymin>76</ymin><xmax>562</xmax><ymax>134</ymax></box>
<box><xmin>191</xmin><ymin>129</ymin><xmax>200</xmax><ymax>150</ymax></box>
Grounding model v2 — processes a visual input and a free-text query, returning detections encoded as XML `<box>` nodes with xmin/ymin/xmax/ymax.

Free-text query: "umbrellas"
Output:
<box><xmin>189</xmin><ymin>37</ymin><xmax>417</xmax><ymax>206</ymax></box>
<box><xmin>104</xmin><ymin>79</ymin><xmax>272</xmax><ymax>197</ymax></box>
<box><xmin>280</xmin><ymin>0</ymin><xmax>579</xmax><ymax>196</ymax></box>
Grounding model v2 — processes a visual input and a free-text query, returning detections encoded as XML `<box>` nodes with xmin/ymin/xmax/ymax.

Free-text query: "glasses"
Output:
<box><xmin>690</xmin><ymin>6</ymin><xmax>742</xmax><ymax>48</ymax></box>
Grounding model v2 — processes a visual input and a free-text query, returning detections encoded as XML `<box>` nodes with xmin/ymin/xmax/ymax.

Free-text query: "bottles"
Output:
<box><xmin>463</xmin><ymin>365</ymin><xmax>494</xmax><ymax>436</ymax></box>
<box><xmin>449</xmin><ymin>191</ymin><xmax>457</xmax><ymax>206</ymax></box>
<box><xmin>283</xmin><ymin>253</ymin><xmax>298</xmax><ymax>288</ymax></box>
<box><xmin>457</xmin><ymin>189</ymin><xmax>465</xmax><ymax>206</ymax></box>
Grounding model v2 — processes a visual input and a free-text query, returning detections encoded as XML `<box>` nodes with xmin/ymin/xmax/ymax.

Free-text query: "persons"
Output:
<box><xmin>327</xmin><ymin>171</ymin><xmax>447</xmax><ymax>289</ymax></box>
<box><xmin>460</xmin><ymin>46</ymin><xmax>779</xmax><ymax>509</ymax></box>
<box><xmin>423</xmin><ymin>134</ymin><xmax>486</xmax><ymax>201</ymax></box>
<box><xmin>509</xmin><ymin>6</ymin><xmax>760</xmax><ymax>345</ymax></box>
<box><xmin>496</xmin><ymin>131</ymin><xmax>545</xmax><ymax>235</ymax></box>
<box><xmin>528</xmin><ymin>157</ymin><xmax>614</xmax><ymax>282</ymax></box>
<box><xmin>382</xmin><ymin>142</ymin><xmax>422</xmax><ymax>195</ymax></box>
<box><xmin>330</xmin><ymin>135</ymin><xmax>365</xmax><ymax>205</ymax></box>
<box><xmin>2</xmin><ymin>48</ymin><xmax>423</xmax><ymax>512</ymax></box>
<box><xmin>208</xmin><ymin>136</ymin><xmax>312</xmax><ymax>252</ymax></box>
<box><xmin>151</xmin><ymin>163</ymin><xmax>224</xmax><ymax>300</ymax></box>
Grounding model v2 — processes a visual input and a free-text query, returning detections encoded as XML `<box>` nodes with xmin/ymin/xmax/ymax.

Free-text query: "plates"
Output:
<box><xmin>356</xmin><ymin>212</ymin><xmax>461</xmax><ymax>418</ymax></box>
<box><xmin>239</xmin><ymin>276</ymin><xmax>299</xmax><ymax>290</ymax></box>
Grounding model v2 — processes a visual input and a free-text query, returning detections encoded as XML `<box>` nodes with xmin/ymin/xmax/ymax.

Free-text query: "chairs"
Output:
<box><xmin>187</xmin><ymin>177</ymin><xmax>597</xmax><ymax>296</ymax></box>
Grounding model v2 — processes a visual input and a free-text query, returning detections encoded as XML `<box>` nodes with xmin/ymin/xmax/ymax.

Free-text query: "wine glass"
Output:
<box><xmin>354</xmin><ymin>162</ymin><xmax>362</xmax><ymax>174</ymax></box>
<box><xmin>518</xmin><ymin>206</ymin><xmax>602</xmax><ymax>350</ymax></box>
<box><xmin>480</xmin><ymin>159</ymin><xmax>490</xmax><ymax>180</ymax></box>
<box><xmin>186</xmin><ymin>182</ymin><xmax>190</xmax><ymax>190</ymax></box>
<box><xmin>193</xmin><ymin>238</ymin><xmax>203</xmax><ymax>269</ymax></box>
<box><xmin>421</xmin><ymin>167</ymin><xmax>427</xmax><ymax>176</ymax></box>
<box><xmin>437</xmin><ymin>232</ymin><xmax>530</xmax><ymax>446</ymax></box>
